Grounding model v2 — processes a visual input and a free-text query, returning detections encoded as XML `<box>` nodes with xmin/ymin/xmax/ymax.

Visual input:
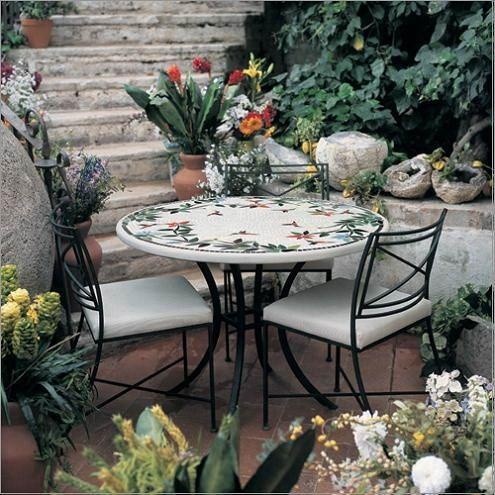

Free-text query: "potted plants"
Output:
<box><xmin>1</xmin><ymin>1</ymin><xmax>80</xmax><ymax>48</ymax></box>
<box><xmin>419</xmin><ymin>281</ymin><xmax>494</xmax><ymax>383</ymax></box>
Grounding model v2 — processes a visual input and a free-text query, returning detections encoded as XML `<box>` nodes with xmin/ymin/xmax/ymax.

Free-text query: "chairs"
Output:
<box><xmin>224</xmin><ymin>164</ymin><xmax>333</xmax><ymax>362</ymax></box>
<box><xmin>49</xmin><ymin>200</ymin><xmax>219</xmax><ymax>434</ymax></box>
<box><xmin>262</xmin><ymin>207</ymin><xmax>459</xmax><ymax>430</ymax></box>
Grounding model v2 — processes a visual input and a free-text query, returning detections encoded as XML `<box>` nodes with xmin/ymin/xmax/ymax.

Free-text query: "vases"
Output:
<box><xmin>235</xmin><ymin>140</ymin><xmax>254</xmax><ymax>158</ymax></box>
<box><xmin>162</xmin><ymin>138</ymin><xmax>181</xmax><ymax>192</ymax></box>
<box><xmin>58</xmin><ymin>219</ymin><xmax>102</xmax><ymax>290</ymax></box>
<box><xmin>2</xmin><ymin>400</ymin><xmax>56</xmax><ymax>493</ymax></box>
<box><xmin>173</xmin><ymin>152</ymin><xmax>209</xmax><ymax>200</ymax></box>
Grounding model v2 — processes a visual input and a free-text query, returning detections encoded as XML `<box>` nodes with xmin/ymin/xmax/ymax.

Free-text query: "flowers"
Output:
<box><xmin>256</xmin><ymin>369</ymin><xmax>495</xmax><ymax>495</ymax></box>
<box><xmin>123</xmin><ymin>52</ymin><xmax>244</xmax><ymax>155</ymax></box>
<box><xmin>190</xmin><ymin>140</ymin><xmax>280</xmax><ymax>201</ymax></box>
<box><xmin>1</xmin><ymin>262</ymin><xmax>112</xmax><ymax>463</ymax></box>
<box><xmin>233</xmin><ymin>50</ymin><xmax>277</xmax><ymax>142</ymax></box>
<box><xmin>1</xmin><ymin>59</ymin><xmax>133</xmax><ymax>227</ymax></box>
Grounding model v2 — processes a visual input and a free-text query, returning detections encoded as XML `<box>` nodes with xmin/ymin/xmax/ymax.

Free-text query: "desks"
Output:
<box><xmin>115</xmin><ymin>195</ymin><xmax>390</xmax><ymax>431</ymax></box>
